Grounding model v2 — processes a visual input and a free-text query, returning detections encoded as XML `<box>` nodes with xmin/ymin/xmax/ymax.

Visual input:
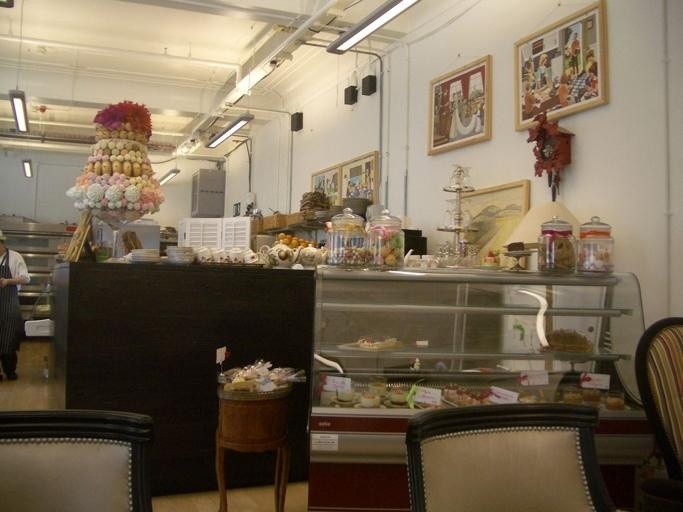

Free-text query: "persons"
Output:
<box><xmin>0</xmin><ymin>229</ymin><xmax>30</xmax><ymax>379</ymax></box>
<box><xmin>522</xmin><ymin>31</ymin><xmax>597</xmax><ymax>113</ymax></box>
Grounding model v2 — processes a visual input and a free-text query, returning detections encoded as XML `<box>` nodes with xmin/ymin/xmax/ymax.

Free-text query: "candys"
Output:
<box><xmin>577</xmin><ymin>248</ymin><xmax>614</xmax><ymax>272</ymax></box>
<box><xmin>368</xmin><ymin>229</ymin><xmax>402</xmax><ymax>266</ymax></box>
<box><xmin>328</xmin><ymin>246</ymin><xmax>367</xmax><ymax>267</ymax></box>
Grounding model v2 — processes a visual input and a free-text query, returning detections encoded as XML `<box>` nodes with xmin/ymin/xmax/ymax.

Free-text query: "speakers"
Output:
<box><xmin>362</xmin><ymin>75</ymin><xmax>376</xmax><ymax>95</ymax></box>
<box><xmin>405</xmin><ymin>236</ymin><xmax>427</xmax><ymax>257</ymax></box>
<box><xmin>291</xmin><ymin>112</ymin><xmax>302</xmax><ymax>131</ymax></box>
<box><xmin>345</xmin><ymin>86</ymin><xmax>358</xmax><ymax>104</ymax></box>
<box><xmin>402</xmin><ymin>229</ymin><xmax>422</xmax><ymax>236</ymax></box>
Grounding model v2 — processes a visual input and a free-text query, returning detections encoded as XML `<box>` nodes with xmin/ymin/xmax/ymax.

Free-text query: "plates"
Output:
<box><xmin>165</xmin><ymin>245</ymin><xmax>195</xmax><ymax>264</ymax></box>
<box><xmin>352</xmin><ymin>403</ymin><xmax>387</xmax><ymax>410</ymax></box>
<box><xmin>130</xmin><ymin>248</ymin><xmax>160</xmax><ymax>263</ymax></box>
<box><xmin>331</xmin><ymin>396</ymin><xmax>357</xmax><ymax>405</ymax></box>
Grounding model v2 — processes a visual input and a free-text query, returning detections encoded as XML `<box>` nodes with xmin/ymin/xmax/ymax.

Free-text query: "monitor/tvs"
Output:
<box><xmin>93</xmin><ymin>217</ymin><xmax>160</xmax><ymax>257</ymax></box>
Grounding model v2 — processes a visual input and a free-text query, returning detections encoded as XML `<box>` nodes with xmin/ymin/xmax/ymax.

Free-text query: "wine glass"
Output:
<box><xmin>447</xmin><ymin>164</ymin><xmax>472</xmax><ymax>189</ymax></box>
<box><xmin>443</xmin><ymin>199</ymin><xmax>472</xmax><ymax>230</ymax></box>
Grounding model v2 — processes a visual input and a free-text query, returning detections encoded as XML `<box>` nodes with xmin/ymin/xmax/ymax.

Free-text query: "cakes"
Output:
<box><xmin>542</xmin><ymin>328</ymin><xmax>597</xmax><ymax>352</ymax></box>
<box><xmin>66</xmin><ymin>101</ymin><xmax>166</xmax><ymax>214</ymax></box>
<box><xmin>481</xmin><ymin>247</ymin><xmax>502</xmax><ymax>267</ymax></box>
<box><xmin>356</xmin><ymin>334</ymin><xmax>399</xmax><ymax>350</ymax></box>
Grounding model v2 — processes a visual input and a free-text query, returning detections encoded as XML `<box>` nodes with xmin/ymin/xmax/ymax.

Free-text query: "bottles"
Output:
<box><xmin>41</xmin><ymin>355</ymin><xmax>51</xmax><ymax>383</ymax></box>
<box><xmin>326</xmin><ymin>207</ymin><xmax>368</xmax><ymax>269</ymax></box>
<box><xmin>577</xmin><ymin>215</ymin><xmax>615</xmax><ymax>274</ymax></box>
<box><xmin>537</xmin><ymin>217</ymin><xmax>577</xmax><ymax>274</ymax></box>
<box><xmin>413</xmin><ymin>357</ymin><xmax>420</xmax><ymax>370</ymax></box>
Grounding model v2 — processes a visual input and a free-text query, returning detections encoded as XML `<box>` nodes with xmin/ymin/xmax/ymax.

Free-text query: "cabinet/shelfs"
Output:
<box><xmin>1</xmin><ymin>223</ymin><xmax>96</xmax><ymax>322</ymax></box>
<box><xmin>306</xmin><ymin>264</ymin><xmax>655</xmax><ymax>512</ymax></box>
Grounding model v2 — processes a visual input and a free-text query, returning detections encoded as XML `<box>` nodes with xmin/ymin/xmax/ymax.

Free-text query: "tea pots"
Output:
<box><xmin>292</xmin><ymin>244</ymin><xmax>327</xmax><ymax>267</ymax></box>
<box><xmin>259</xmin><ymin>240</ymin><xmax>302</xmax><ymax>270</ymax></box>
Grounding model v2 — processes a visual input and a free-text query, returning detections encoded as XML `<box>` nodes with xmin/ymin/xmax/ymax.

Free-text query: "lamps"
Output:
<box><xmin>326</xmin><ymin>1</ymin><xmax>420</xmax><ymax>55</ymax></box>
<box><xmin>205</xmin><ymin>72</ymin><xmax>255</xmax><ymax>149</ymax></box>
<box><xmin>9</xmin><ymin>0</ymin><xmax>30</xmax><ymax>133</ymax></box>
<box><xmin>159</xmin><ymin>134</ymin><xmax>181</xmax><ymax>186</ymax></box>
<box><xmin>22</xmin><ymin>149</ymin><xmax>33</xmax><ymax>178</ymax></box>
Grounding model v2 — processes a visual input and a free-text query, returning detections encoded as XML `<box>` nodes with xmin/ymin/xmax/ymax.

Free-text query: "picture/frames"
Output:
<box><xmin>457</xmin><ymin>179</ymin><xmax>531</xmax><ymax>259</ymax></box>
<box><xmin>341</xmin><ymin>150</ymin><xmax>379</xmax><ymax>216</ymax></box>
<box><xmin>429</xmin><ymin>53</ymin><xmax>494</xmax><ymax>157</ymax></box>
<box><xmin>513</xmin><ymin>1</ymin><xmax>610</xmax><ymax>132</ymax></box>
<box><xmin>311</xmin><ymin>162</ymin><xmax>339</xmax><ymax>216</ymax></box>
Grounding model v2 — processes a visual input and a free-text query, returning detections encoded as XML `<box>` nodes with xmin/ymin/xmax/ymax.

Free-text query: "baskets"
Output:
<box><xmin>177</xmin><ymin>217</ymin><xmax>252</xmax><ymax>251</ymax></box>
<box><xmin>251</xmin><ymin>198</ymin><xmax>372</xmax><ymax>232</ymax></box>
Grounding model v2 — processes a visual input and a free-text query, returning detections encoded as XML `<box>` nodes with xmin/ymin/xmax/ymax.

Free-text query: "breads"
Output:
<box><xmin>273</xmin><ymin>233</ymin><xmax>309</xmax><ymax>248</ymax></box>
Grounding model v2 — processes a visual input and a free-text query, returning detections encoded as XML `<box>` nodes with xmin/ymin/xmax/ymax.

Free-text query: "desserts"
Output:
<box><xmin>581</xmin><ymin>389</ymin><xmax>601</xmax><ymax>409</ymax></box>
<box><xmin>606</xmin><ymin>396</ymin><xmax>625</xmax><ymax>411</ymax></box>
<box><xmin>444</xmin><ymin>386</ymin><xmax>487</xmax><ymax>407</ymax></box>
<box><xmin>519</xmin><ymin>395</ymin><xmax>537</xmax><ymax>404</ymax></box>
<box><xmin>369</xmin><ymin>382</ymin><xmax>385</xmax><ymax>396</ymax></box>
<box><xmin>390</xmin><ymin>391</ymin><xmax>407</xmax><ymax>405</ymax></box>
<box><xmin>564</xmin><ymin>392</ymin><xmax>583</xmax><ymax>404</ymax></box>
<box><xmin>360</xmin><ymin>394</ymin><xmax>380</xmax><ymax>407</ymax></box>
<box><xmin>336</xmin><ymin>387</ymin><xmax>353</xmax><ymax>402</ymax></box>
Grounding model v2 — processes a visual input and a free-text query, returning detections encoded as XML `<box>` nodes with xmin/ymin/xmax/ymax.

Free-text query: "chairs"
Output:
<box><xmin>0</xmin><ymin>410</ymin><xmax>154</xmax><ymax>512</ymax></box>
<box><xmin>405</xmin><ymin>404</ymin><xmax>618</xmax><ymax>511</ymax></box>
<box><xmin>635</xmin><ymin>318</ymin><xmax>683</xmax><ymax>511</ymax></box>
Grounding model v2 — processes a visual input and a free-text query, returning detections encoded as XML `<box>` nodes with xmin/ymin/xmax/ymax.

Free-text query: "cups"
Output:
<box><xmin>334</xmin><ymin>386</ymin><xmax>354</xmax><ymax>402</ymax></box>
<box><xmin>95</xmin><ymin>240</ymin><xmax>108</xmax><ymax>259</ymax></box>
<box><xmin>360</xmin><ymin>395</ymin><xmax>379</xmax><ymax>408</ymax></box>
<box><xmin>560</xmin><ymin>387</ymin><xmax>626</xmax><ymax>410</ymax></box>
<box><xmin>365</xmin><ymin>212</ymin><xmax>404</xmax><ymax>269</ymax></box>
<box><xmin>195</xmin><ymin>247</ymin><xmax>257</xmax><ymax>264</ymax></box>
<box><xmin>408</xmin><ymin>254</ymin><xmax>437</xmax><ymax>270</ymax></box>
